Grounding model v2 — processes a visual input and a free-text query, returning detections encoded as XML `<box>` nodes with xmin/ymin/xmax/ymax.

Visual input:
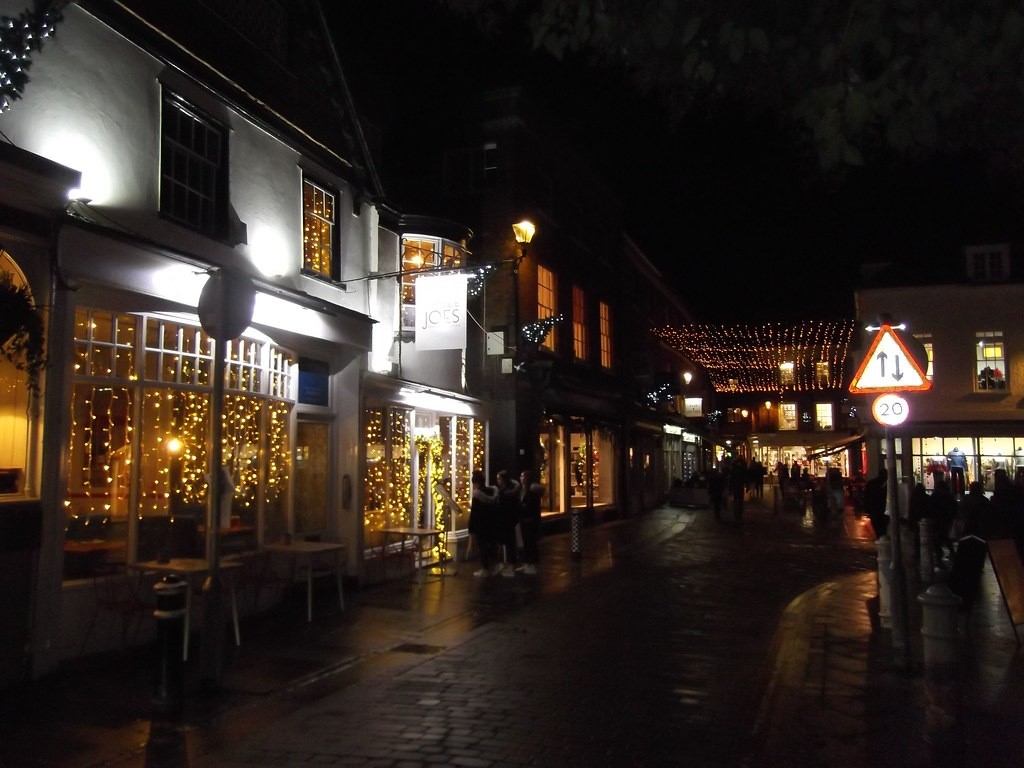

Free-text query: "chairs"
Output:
<box><xmin>77</xmin><ymin>572</ymin><xmax>156</xmax><ymax>667</ymax></box>
<box><xmin>239</xmin><ymin>550</ymin><xmax>294</xmax><ymax>618</ymax></box>
<box><xmin>370</xmin><ymin>533</ymin><xmax>413</xmax><ymax>591</ymax></box>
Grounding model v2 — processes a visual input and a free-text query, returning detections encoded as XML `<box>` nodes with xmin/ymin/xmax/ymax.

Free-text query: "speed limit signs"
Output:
<box><xmin>871</xmin><ymin>392</ymin><xmax>914</xmax><ymax>428</ymax></box>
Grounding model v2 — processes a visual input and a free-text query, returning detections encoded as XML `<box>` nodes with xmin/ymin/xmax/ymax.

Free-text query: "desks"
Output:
<box><xmin>268</xmin><ymin>539</ymin><xmax>347</xmax><ymax>623</ymax></box>
<box><xmin>128</xmin><ymin>558</ymin><xmax>243</xmax><ymax>662</ymax></box>
<box><xmin>381</xmin><ymin>527</ymin><xmax>444</xmax><ymax>590</ymax></box>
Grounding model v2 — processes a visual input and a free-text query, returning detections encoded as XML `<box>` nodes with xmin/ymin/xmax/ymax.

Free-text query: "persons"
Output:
<box><xmin>993</xmin><ymin>446</ymin><xmax>1024</xmax><ymax>483</ymax></box>
<box><xmin>928</xmin><ymin>448</ymin><xmax>968</xmax><ymax>500</ymax></box>
<box><xmin>496</xmin><ymin>471</ymin><xmax>522</xmax><ymax>576</ymax></box>
<box><xmin>467</xmin><ymin>470</ymin><xmax>499</xmax><ymax>577</ymax></box>
<box><xmin>861</xmin><ymin>469</ymin><xmax>1024</xmax><ymax>559</ymax></box>
<box><xmin>709</xmin><ymin>456</ymin><xmax>815</xmax><ymax>520</ymax></box>
<box><xmin>516</xmin><ymin>471</ymin><xmax>546</xmax><ymax>574</ymax></box>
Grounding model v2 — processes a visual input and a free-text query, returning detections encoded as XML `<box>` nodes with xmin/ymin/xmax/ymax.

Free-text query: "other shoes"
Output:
<box><xmin>492</xmin><ymin>563</ymin><xmax>504</xmax><ymax>577</ymax></box>
<box><xmin>502</xmin><ymin>569</ymin><xmax>515</xmax><ymax>577</ymax></box>
<box><xmin>524</xmin><ymin>564</ymin><xmax>537</xmax><ymax>574</ymax></box>
<box><xmin>515</xmin><ymin>563</ymin><xmax>528</xmax><ymax>571</ymax></box>
<box><xmin>473</xmin><ymin>568</ymin><xmax>488</xmax><ymax>578</ymax></box>
